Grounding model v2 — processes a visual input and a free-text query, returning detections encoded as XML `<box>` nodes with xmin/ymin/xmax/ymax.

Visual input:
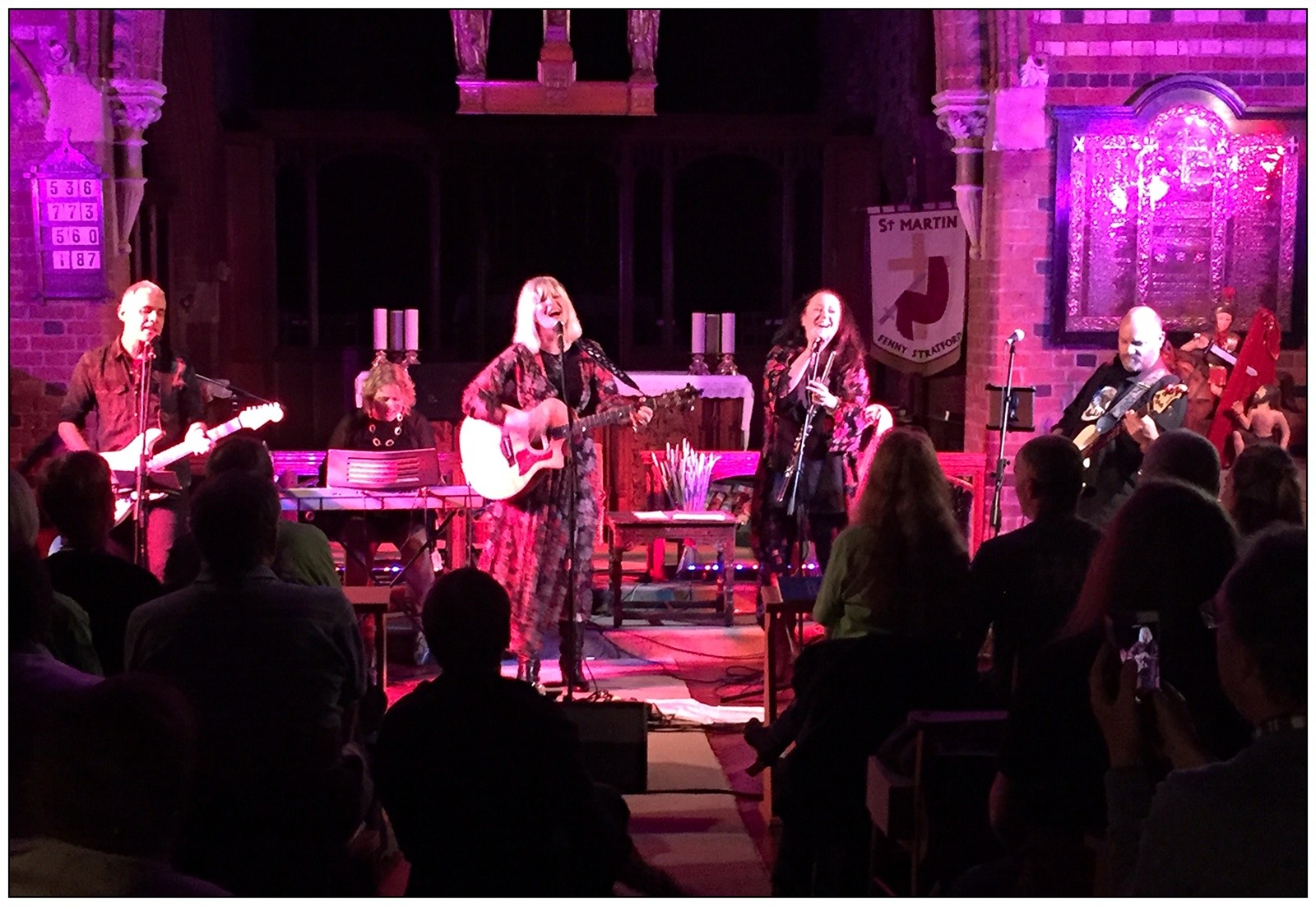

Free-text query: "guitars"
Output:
<box><xmin>460</xmin><ymin>373</ymin><xmax>706</xmax><ymax>501</ymax></box>
<box><xmin>98</xmin><ymin>398</ymin><xmax>286</xmax><ymax>534</ymax></box>
<box><xmin>1072</xmin><ymin>381</ymin><xmax>1192</xmax><ymax>471</ymax></box>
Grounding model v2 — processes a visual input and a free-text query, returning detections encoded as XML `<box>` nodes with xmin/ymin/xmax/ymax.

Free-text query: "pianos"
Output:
<box><xmin>293</xmin><ymin>445</ymin><xmax>485</xmax><ymax>638</ymax></box>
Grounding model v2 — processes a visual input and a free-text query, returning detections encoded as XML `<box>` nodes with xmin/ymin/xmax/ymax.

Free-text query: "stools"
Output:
<box><xmin>339</xmin><ymin>585</ymin><xmax>392</xmax><ymax>701</ymax></box>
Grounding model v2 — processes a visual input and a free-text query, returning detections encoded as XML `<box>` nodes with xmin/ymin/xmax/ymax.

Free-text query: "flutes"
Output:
<box><xmin>778</xmin><ymin>351</ymin><xmax>842</xmax><ymax>504</ymax></box>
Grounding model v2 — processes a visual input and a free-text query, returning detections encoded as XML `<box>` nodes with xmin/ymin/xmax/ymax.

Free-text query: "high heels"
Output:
<box><xmin>744</xmin><ymin>717</ymin><xmax>783</xmax><ymax>777</ymax></box>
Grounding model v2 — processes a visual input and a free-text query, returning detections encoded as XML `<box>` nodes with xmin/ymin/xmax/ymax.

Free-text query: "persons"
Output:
<box><xmin>743</xmin><ymin>423</ymin><xmax>973</xmax><ymax>776</ymax></box>
<box><xmin>1049</xmin><ymin>307</ymin><xmax>1189</xmax><ymax>519</ymax></box>
<box><xmin>8</xmin><ymin>550</ymin><xmax>108</xmax><ymax>841</ymax></box>
<box><xmin>164</xmin><ymin>432</ymin><xmax>344</xmax><ymax>590</ymax></box>
<box><xmin>328</xmin><ymin>358</ymin><xmax>436</xmax><ymax>682</ymax></box>
<box><xmin>1181</xmin><ymin>286</ymin><xmax>1243</xmax><ymax>400</ymax></box>
<box><xmin>920</xmin><ymin>436</ymin><xmax>1104</xmax><ymax>897</ymax></box>
<box><xmin>58</xmin><ymin>280</ymin><xmax>207</xmax><ymax>585</ymax></box>
<box><xmin>1027</xmin><ymin>428</ymin><xmax>1308</xmax><ymax>898</ymax></box>
<box><xmin>37</xmin><ymin>450</ymin><xmax>167</xmax><ymax>668</ymax></box>
<box><xmin>749</xmin><ymin>289</ymin><xmax>872</xmax><ymax>628</ymax></box>
<box><xmin>1231</xmin><ymin>383</ymin><xmax>1290</xmax><ymax>456</ymax></box>
<box><xmin>370</xmin><ymin>566</ymin><xmax>633</xmax><ymax>898</ymax></box>
<box><xmin>460</xmin><ymin>277</ymin><xmax>656</xmax><ymax>695</ymax></box>
<box><xmin>117</xmin><ymin>467</ymin><xmax>366</xmax><ymax>898</ymax></box>
<box><xmin>9</xmin><ymin>467</ymin><xmax>100</xmax><ymax>676</ymax></box>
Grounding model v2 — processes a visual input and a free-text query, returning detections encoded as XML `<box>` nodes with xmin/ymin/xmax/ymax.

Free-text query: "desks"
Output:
<box><xmin>604</xmin><ymin>507</ymin><xmax>741</xmax><ymax>631</ymax></box>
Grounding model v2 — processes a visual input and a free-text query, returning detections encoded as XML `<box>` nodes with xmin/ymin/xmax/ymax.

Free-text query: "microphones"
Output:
<box><xmin>811</xmin><ymin>337</ymin><xmax>825</xmax><ymax>353</ymax></box>
<box><xmin>1005</xmin><ymin>328</ymin><xmax>1025</xmax><ymax>345</ymax></box>
<box><xmin>143</xmin><ymin>328</ymin><xmax>157</xmax><ymax>359</ymax></box>
<box><xmin>553</xmin><ymin>318</ymin><xmax>564</xmax><ymax>349</ymax></box>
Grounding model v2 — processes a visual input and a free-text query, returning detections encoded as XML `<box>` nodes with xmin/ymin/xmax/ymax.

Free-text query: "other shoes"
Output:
<box><xmin>363</xmin><ymin>617</ymin><xmax>429</xmax><ymax>686</ymax></box>
<box><xmin>774</xmin><ymin>634</ymin><xmax>790</xmax><ymax>683</ymax></box>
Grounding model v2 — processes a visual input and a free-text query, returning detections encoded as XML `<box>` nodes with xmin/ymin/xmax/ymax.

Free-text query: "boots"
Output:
<box><xmin>515</xmin><ymin>621</ymin><xmax>590</xmax><ymax>693</ymax></box>
